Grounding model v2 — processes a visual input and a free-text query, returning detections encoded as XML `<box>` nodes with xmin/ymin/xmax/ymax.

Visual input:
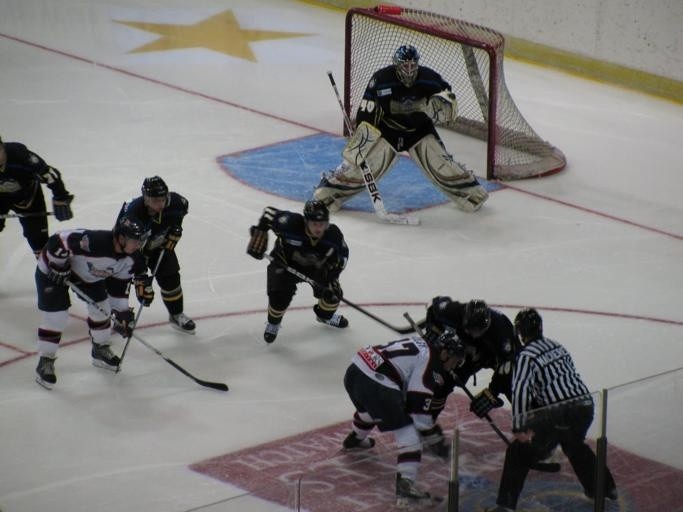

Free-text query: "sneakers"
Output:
<box><xmin>396</xmin><ymin>478</ymin><xmax>431</xmax><ymax>499</ymax></box>
<box><xmin>343</xmin><ymin>434</ymin><xmax>376</xmax><ymax>449</ymax></box>
<box><xmin>583</xmin><ymin>489</ymin><xmax>618</xmax><ymax>501</ymax></box>
<box><xmin>264</xmin><ymin>321</ymin><xmax>281</xmax><ymax>343</ymax></box>
<box><xmin>313</xmin><ymin>305</ymin><xmax>348</xmax><ymax>328</ymax></box>
<box><xmin>36</xmin><ymin>356</ymin><xmax>57</xmax><ymax>389</ymax></box>
<box><xmin>169</xmin><ymin>313</ymin><xmax>197</xmax><ymax>334</ymax></box>
<box><xmin>91</xmin><ymin>340</ymin><xmax>120</xmax><ymax>371</ymax></box>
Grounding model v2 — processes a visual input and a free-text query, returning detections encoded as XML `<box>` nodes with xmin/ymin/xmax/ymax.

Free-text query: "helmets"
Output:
<box><xmin>115</xmin><ymin>209</ymin><xmax>151</xmax><ymax>241</ymax></box>
<box><xmin>303</xmin><ymin>199</ymin><xmax>329</xmax><ymax>222</ymax></box>
<box><xmin>393</xmin><ymin>44</ymin><xmax>420</xmax><ymax>84</ymax></box>
<box><xmin>140</xmin><ymin>176</ymin><xmax>168</xmax><ymax>198</ymax></box>
<box><xmin>431</xmin><ymin>297</ymin><xmax>543</xmax><ymax>372</ymax></box>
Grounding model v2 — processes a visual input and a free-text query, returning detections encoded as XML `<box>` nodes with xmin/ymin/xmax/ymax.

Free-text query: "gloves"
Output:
<box><xmin>469</xmin><ymin>387</ymin><xmax>497</xmax><ymax>419</ymax></box>
<box><xmin>245</xmin><ymin>228</ymin><xmax>268</xmax><ymax>260</ymax></box>
<box><xmin>52</xmin><ymin>194</ymin><xmax>74</xmax><ymax>222</ymax></box>
<box><xmin>313</xmin><ymin>269</ymin><xmax>330</xmax><ymax>299</ymax></box>
<box><xmin>430</xmin><ymin>439</ymin><xmax>451</xmax><ymax>464</ymax></box>
<box><xmin>111</xmin><ymin>308</ymin><xmax>135</xmax><ymax>338</ymax></box>
<box><xmin>134</xmin><ymin>274</ymin><xmax>155</xmax><ymax>307</ymax></box>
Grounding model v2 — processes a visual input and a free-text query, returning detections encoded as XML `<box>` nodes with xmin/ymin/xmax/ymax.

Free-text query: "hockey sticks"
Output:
<box><xmin>264</xmin><ymin>252</ymin><xmax>427</xmax><ymax>334</ymax></box>
<box><xmin>64</xmin><ymin>278</ymin><xmax>228</xmax><ymax>392</ymax></box>
<box><xmin>402</xmin><ymin>311</ymin><xmax>561</xmax><ymax>473</ymax></box>
<box><xmin>113</xmin><ymin>249</ymin><xmax>167</xmax><ymax>374</ymax></box>
<box><xmin>327</xmin><ymin>71</ymin><xmax>421</xmax><ymax>225</ymax></box>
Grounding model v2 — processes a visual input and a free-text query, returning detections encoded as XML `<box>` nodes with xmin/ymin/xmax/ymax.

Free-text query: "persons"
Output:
<box><xmin>343</xmin><ymin>330</ymin><xmax>466</xmax><ymax>500</ymax></box>
<box><xmin>314</xmin><ymin>45</ymin><xmax>490</xmax><ymax>215</ymax></box>
<box><xmin>36</xmin><ymin>212</ymin><xmax>151</xmax><ymax>381</ymax></box>
<box><xmin>486</xmin><ymin>309</ymin><xmax>615</xmax><ymax>511</ymax></box>
<box><xmin>0</xmin><ymin>139</ymin><xmax>73</xmax><ymax>259</ymax></box>
<box><xmin>116</xmin><ymin>176</ymin><xmax>196</xmax><ymax>332</ymax></box>
<box><xmin>426</xmin><ymin>294</ymin><xmax>522</xmax><ymax>422</ymax></box>
<box><xmin>247</xmin><ymin>199</ymin><xmax>347</xmax><ymax>343</ymax></box>
